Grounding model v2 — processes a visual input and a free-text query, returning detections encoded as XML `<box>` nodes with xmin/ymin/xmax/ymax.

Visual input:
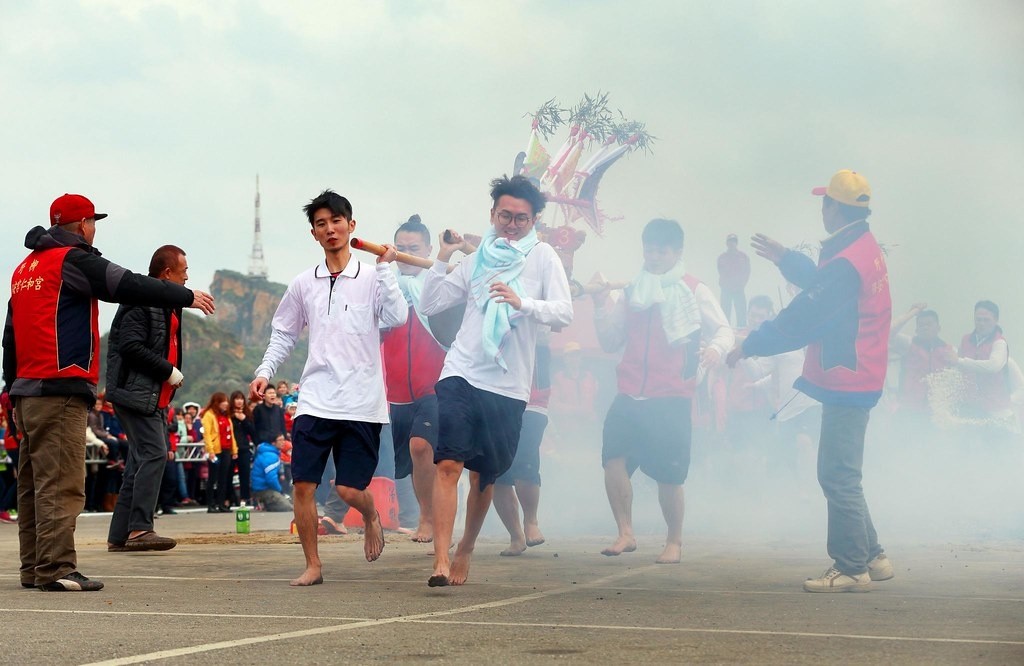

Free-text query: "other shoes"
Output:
<box><xmin>161</xmin><ymin>510</ymin><xmax>177</xmax><ymax>514</ymax></box>
<box><xmin>321</xmin><ymin>516</ymin><xmax>347</xmax><ymax>534</ymax></box>
<box><xmin>207</xmin><ymin>505</ymin><xmax>232</xmax><ymax>513</ymax></box>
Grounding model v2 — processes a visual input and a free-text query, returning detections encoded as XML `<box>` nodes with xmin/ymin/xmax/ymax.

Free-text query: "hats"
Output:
<box><xmin>727</xmin><ymin>234</ymin><xmax>736</xmax><ymax>240</ymax></box>
<box><xmin>812</xmin><ymin>170</ymin><xmax>871</xmax><ymax>207</ymax></box>
<box><xmin>50</xmin><ymin>193</ymin><xmax>108</xmax><ymax>226</ymax></box>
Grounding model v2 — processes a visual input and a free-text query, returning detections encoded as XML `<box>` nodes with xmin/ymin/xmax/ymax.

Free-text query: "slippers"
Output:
<box><xmin>107</xmin><ymin>542</ymin><xmax>125</xmax><ymax>551</ymax></box>
<box><xmin>125</xmin><ymin>531</ymin><xmax>176</xmax><ymax>550</ymax></box>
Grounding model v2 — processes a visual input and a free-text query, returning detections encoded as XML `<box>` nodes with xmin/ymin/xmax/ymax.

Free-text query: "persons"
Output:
<box><xmin>734</xmin><ymin>298</ymin><xmax>1012</xmax><ymax>428</ymax></box>
<box><xmin>586</xmin><ymin>217</ymin><xmax>735</xmax><ymax>566</ymax></box>
<box><xmin>717</xmin><ymin>233</ymin><xmax>752</xmax><ymax>336</ymax></box>
<box><xmin>372</xmin><ymin>213</ymin><xmax>446</xmax><ymax>544</ymax></box>
<box><xmin>725</xmin><ymin>168</ymin><xmax>896</xmax><ymax>593</ymax></box>
<box><xmin>247</xmin><ymin>188</ymin><xmax>407</xmax><ymax>588</ymax></box>
<box><xmin>0</xmin><ymin>379</ymin><xmax>353</xmax><ymax>533</ymax></box>
<box><xmin>416</xmin><ymin>172</ymin><xmax>575</xmax><ymax>590</ymax></box>
<box><xmin>489</xmin><ymin>331</ymin><xmax>549</xmax><ymax>557</ymax></box>
<box><xmin>104</xmin><ymin>245</ymin><xmax>189</xmax><ymax>552</ymax></box>
<box><xmin>1</xmin><ymin>192</ymin><xmax>217</xmax><ymax>592</ymax></box>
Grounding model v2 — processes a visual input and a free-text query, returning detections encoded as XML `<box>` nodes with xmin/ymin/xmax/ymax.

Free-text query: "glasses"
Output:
<box><xmin>495</xmin><ymin>210</ymin><xmax>532</xmax><ymax>228</ymax></box>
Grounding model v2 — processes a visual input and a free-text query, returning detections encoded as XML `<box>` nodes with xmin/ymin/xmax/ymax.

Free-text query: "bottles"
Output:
<box><xmin>236</xmin><ymin>501</ymin><xmax>250</xmax><ymax>534</ymax></box>
<box><xmin>205</xmin><ymin>453</ymin><xmax>219</xmax><ymax>465</ymax></box>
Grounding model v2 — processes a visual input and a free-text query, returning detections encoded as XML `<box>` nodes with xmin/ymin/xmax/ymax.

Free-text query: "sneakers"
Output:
<box><xmin>803</xmin><ymin>552</ymin><xmax>894</xmax><ymax>593</ymax></box>
<box><xmin>38</xmin><ymin>571</ymin><xmax>103</xmax><ymax>590</ymax></box>
<box><xmin>22</xmin><ymin>577</ymin><xmax>35</xmax><ymax>587</ymax></box>
